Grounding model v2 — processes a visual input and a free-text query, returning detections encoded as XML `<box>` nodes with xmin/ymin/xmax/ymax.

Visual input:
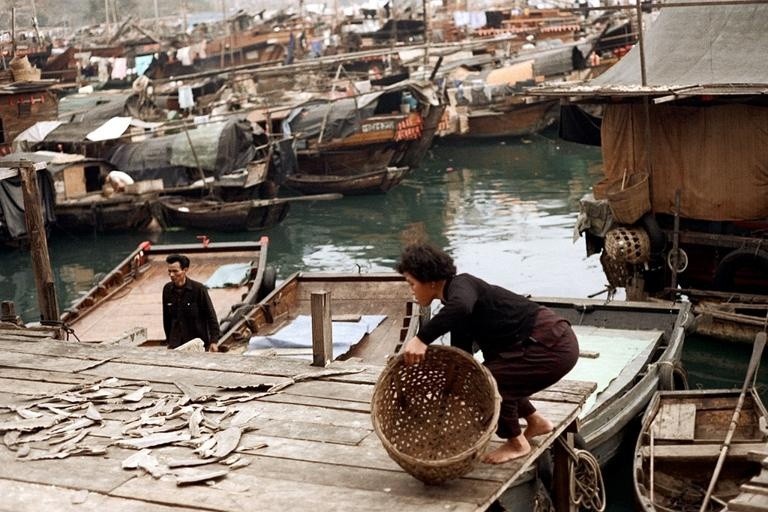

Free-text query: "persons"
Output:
<box><xmin>162</xmin><ymin>254</ymin><xmax>220</xmax><ymax>353</ymax></box>
<box><xmin>393</xmin><ymin>238</ymin><xmax>580</xmax><ymax>466</ymax></box>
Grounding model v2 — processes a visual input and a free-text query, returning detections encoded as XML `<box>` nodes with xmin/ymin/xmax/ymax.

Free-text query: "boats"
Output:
<box><xmin>61</xmin><ymin>236</ymin><xmax>269</xmax><ymax>353</ymax></box>
<box><xmin>633</xmin><ymin>387</ymin><xmax>768</xmax><ymax>512</ymax></box>
<box><xmin>474</xmin><ymin>293</ymin><xmax>692</xmax><ymax>512</ymax></box>
<box><xmin>510</xmin><ymin>0</ymin><xmax>768</xmax><ymax>405</ymax></box>
<box><xmin>217</xmin><ymin>271</ymin><xmax>421</xmax><ymax>371</ymax></box>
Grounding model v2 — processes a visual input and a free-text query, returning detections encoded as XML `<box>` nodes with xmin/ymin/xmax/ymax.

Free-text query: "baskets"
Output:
<box><xmin>372</xmin><ymin>344</ymin><xmax>502</xmax><ymax>486</ymax></box>
<box><xmin>594</xmin><ymin>171</ymin><xmax>652</xmax><ymax>287</ymax></box>
